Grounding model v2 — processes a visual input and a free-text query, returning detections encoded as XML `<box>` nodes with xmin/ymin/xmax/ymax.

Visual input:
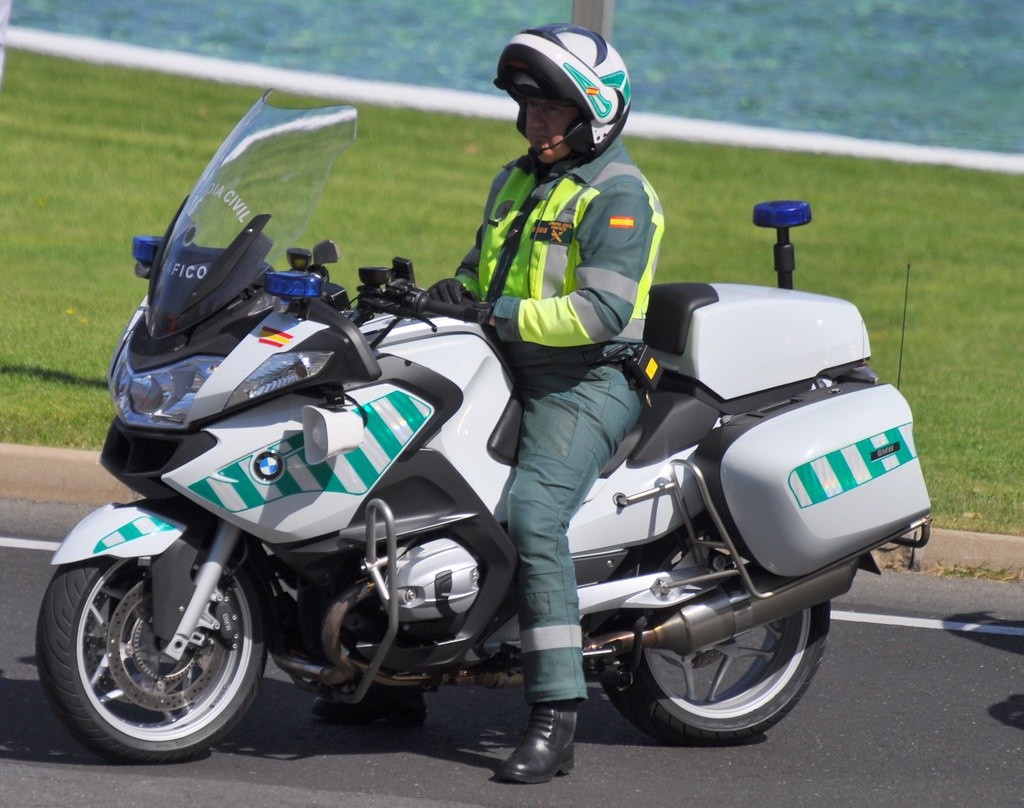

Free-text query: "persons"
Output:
<box><xmin>426</xmin><ymin>19</ymin><xmax>670</xmax><ymax>784</ymax></box>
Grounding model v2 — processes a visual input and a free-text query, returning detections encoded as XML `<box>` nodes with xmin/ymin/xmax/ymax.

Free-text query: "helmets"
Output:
<box><xmin>492</xmin><ymin>22</ymin><xmax>631</xmax><ymax>162</ymax></box>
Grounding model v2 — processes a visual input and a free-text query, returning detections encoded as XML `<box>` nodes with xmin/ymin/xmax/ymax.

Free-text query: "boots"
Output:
<box><xmin>495</xmin><ymin>700</ymin><xmax>578</xmax><ymax>783</ymax></box>
<box><xmin>311</xmin><ymin>682</ymin><xmax>426</xmax><ymax>727</ymax></box>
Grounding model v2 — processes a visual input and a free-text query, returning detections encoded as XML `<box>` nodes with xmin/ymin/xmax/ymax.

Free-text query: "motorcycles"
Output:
<box><xmin>30</xmin><ymin>87</ymin><xmax>935</xmax><ymax>768</ymax></box>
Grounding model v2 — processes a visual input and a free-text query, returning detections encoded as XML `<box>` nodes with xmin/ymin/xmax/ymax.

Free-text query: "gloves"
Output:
<box><xmin>426</xmin><ymin>278</ymin><xmax>478</xmax><ymax>306</ymax></box>
